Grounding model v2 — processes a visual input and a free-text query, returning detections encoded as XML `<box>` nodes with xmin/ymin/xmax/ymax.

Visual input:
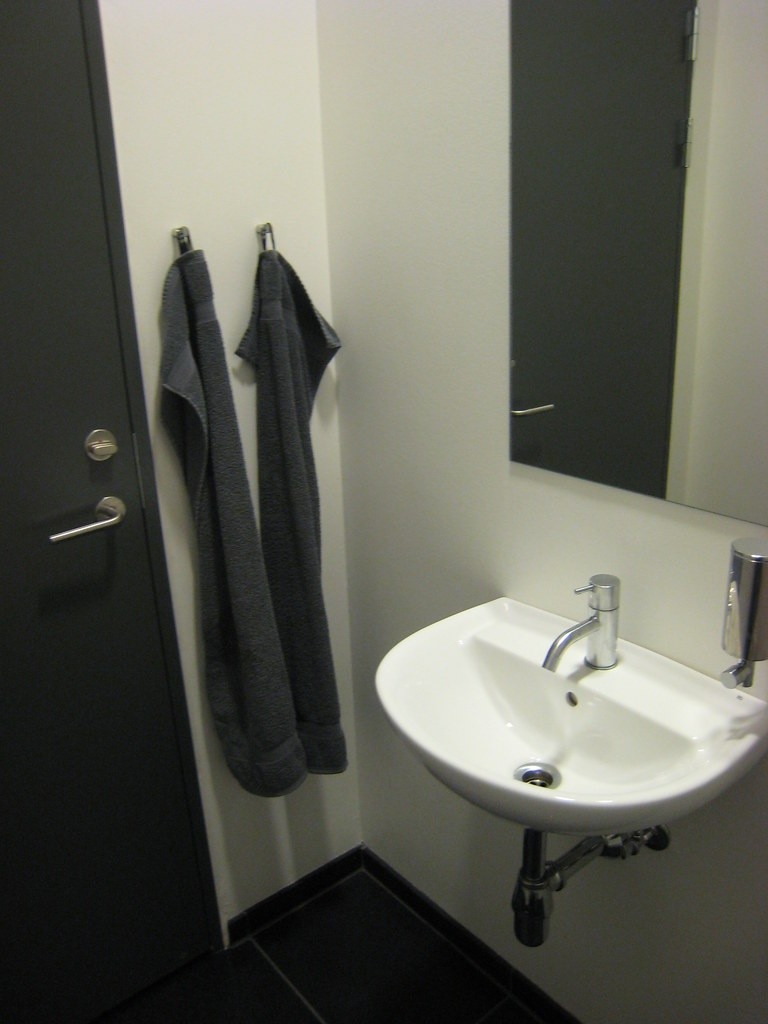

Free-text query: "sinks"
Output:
<box><xmin>375</xmin><ymin>595</ymin><xmax>767</xmax><ymax>838</ymax></box>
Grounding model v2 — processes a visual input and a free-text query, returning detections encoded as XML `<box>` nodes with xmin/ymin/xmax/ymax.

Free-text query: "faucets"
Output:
<box><xmin>541</xmin><ymin>573</ymin><xmax>620</xmax><ymax>673</ymax></box>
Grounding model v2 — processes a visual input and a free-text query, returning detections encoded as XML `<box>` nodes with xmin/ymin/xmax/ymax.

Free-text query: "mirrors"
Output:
<box><xmin>508</xmin><ymin>0</ymin><xmax>768</xmax><ymax>523</ymax></box>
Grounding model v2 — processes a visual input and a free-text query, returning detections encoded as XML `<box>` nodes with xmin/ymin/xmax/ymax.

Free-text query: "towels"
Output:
<box><xmin>159</xmin><ymin>223</ymin><xmax>349</xmax><ymax>799</ymax></box>
